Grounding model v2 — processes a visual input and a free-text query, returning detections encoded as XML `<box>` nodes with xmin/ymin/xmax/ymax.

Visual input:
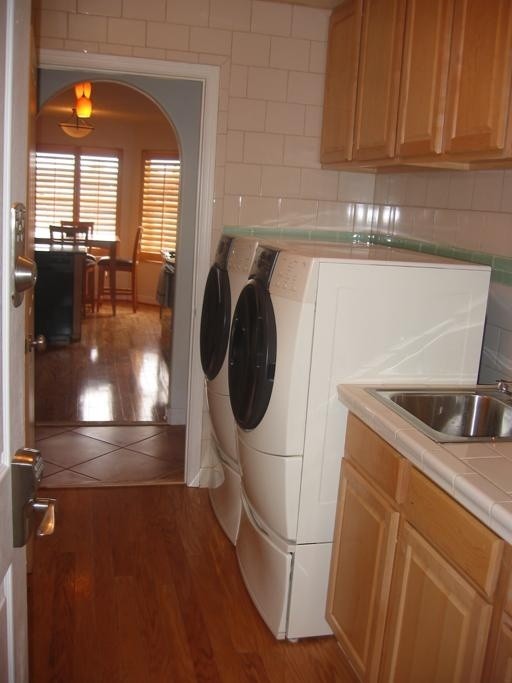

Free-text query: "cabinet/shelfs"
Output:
<box><xmin>395</xmin><ymin>0</ymin><xmax>512</xmax><ymax>171</ymax></box>
<box><xmin>319</xmin><ymin>0</ymin><xmax>467</xmax><ymax>175</ymax></box>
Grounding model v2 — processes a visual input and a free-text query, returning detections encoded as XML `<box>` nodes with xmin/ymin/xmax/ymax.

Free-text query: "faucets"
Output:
<box><xmin>496</xmin><ymin>382</ymin><xmax>512</xmax><ymax>397</ymax></box>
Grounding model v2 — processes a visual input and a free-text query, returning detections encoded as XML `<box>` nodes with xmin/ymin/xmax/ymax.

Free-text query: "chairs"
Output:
<box><xmin>61</xmin><ymin>221</ymin><xmax>94</xmax><ymax>255</ymax></box>
<box><xmin>49</xmin><ymin>225</ymin><xmax>96</xmax><ymax>319</ymax></box>
<box><xmin>97</xmin><ymin>227</ymin><xmax>144</xmax><ymax>312</ymax></box>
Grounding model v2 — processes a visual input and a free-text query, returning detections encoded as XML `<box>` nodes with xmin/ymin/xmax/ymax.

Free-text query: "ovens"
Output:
<box><xmin>34</xmin><ymin>243</ymin><xmax>97</xmax><ymax>346</ymax></box>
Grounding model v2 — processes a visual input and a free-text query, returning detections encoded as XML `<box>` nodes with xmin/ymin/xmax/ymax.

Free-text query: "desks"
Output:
<box><xmin>35</xmin><ymin>231</ymin><xmax>121</xmax><ymax>315</ymax></box>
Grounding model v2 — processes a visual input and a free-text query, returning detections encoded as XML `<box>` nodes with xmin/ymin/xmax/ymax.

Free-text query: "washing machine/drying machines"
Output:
<box><xmin>228</xmin><ymin>241</ymin><xmax>492</xmax><ymax>643</ymax></box>
<box><xmin>199</xmin><ymin>233</ymin><xmax>259</xmax><ymax>540</ymax></box>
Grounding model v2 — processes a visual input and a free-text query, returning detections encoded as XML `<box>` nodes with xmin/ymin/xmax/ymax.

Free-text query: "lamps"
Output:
<box><xmin>75</xmin><ymin>82</ymin><xmax>92</xmax><ymax>118</ymax></box>
<box><xmin>58</xmin><ymin>108</ymin><xmax>94</xmax><ymax>138</ymax></box>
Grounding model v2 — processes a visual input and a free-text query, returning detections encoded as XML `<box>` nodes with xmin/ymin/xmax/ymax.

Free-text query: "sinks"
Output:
<box><xmin>365</xmin><ymin>387</ymin><xmax>512</xmax><ymax>443</ymax></box>
